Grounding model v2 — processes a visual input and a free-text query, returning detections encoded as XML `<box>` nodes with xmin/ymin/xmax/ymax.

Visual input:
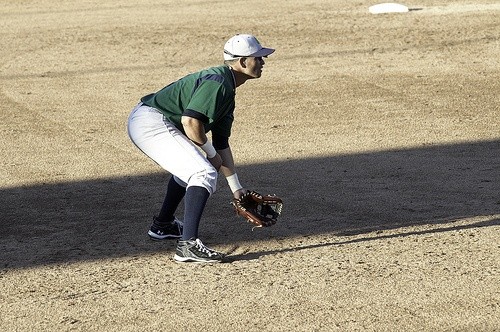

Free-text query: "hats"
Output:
<box><xmin>223</xmin><ymin>33</ymin><xmax>275</xmax><ymax>62</ymax></box>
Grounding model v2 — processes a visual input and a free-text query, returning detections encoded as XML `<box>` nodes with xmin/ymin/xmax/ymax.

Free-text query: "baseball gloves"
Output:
<box><xmin>233</xmin><ymin>190</ymin><xmax>284</xmax><ymax>228</ymax></box>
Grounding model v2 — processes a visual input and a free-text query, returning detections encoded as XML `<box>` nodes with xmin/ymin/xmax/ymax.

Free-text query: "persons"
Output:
<box><xmin>127</xmin><ymin>33</ymin><xmax>276</xmax><ymax>264</ymax></box>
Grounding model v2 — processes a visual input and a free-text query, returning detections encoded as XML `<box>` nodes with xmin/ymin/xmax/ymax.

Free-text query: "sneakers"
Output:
<box><xmin>147</xmin><ymin>216</ymin><xmax>184</xmax><ymax>240</ymax></box>
<box><xmin>174</xmin><ymin>237</ymin><xmax>225</xmax><ymax>263</ymax></box>
